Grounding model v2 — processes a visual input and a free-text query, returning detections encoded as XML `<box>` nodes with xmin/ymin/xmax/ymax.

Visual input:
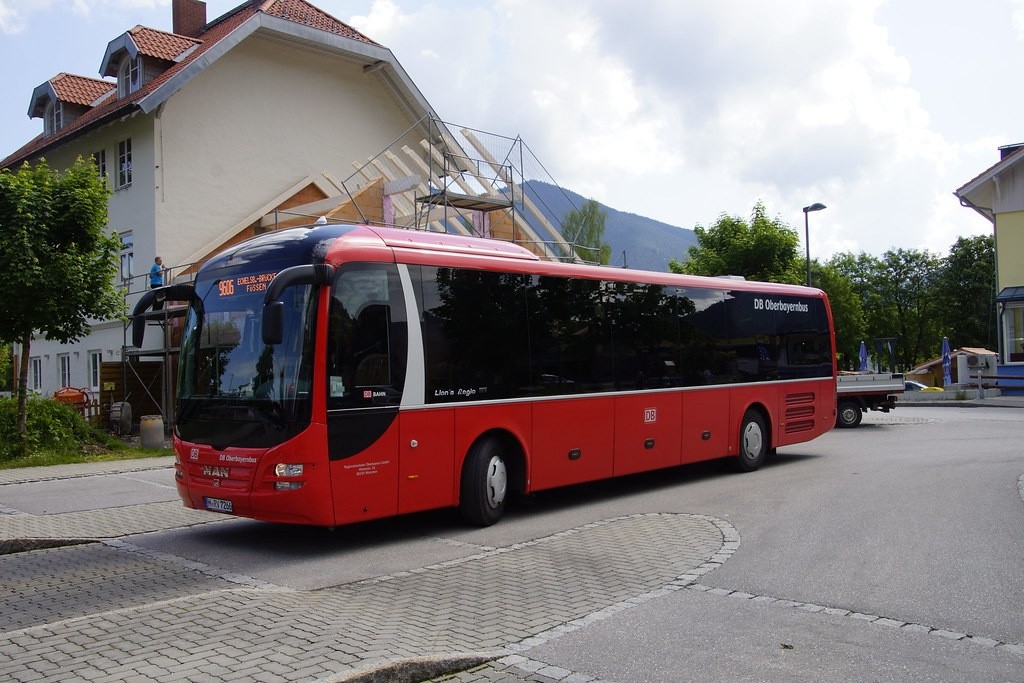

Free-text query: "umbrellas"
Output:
<box><xmin>943</xmin><ymin>338</ymin><xmax>951</xmax><ymax>386</ymax></box>
<box><xmin>859</xmin><ymin>342</ymin><xmax>867</xmax><ymax>371</ymax></box>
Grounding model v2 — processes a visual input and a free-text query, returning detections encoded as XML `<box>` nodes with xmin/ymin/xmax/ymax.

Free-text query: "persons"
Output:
<box><xmin>149</xmin><ymin>257</ymin><xmax>165</xmax><ymax>310</ymax></box>
<box><xmin>341</xmin><ymin>322</ymin><xmax>384</xmax><ymax>395</ymax></box>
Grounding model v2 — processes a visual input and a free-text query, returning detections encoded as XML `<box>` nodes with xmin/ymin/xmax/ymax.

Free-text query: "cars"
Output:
<box><xmin>904</xmin><ymin>380</ymin><xmax>928</xmax><ymax>392</ymax></box>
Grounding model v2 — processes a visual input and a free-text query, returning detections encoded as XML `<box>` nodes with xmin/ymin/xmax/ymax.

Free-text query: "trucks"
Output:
<box><xmin>835</xmin><ymin>373</ymin><xmax>905</xmax><ymax>428</ymax></box>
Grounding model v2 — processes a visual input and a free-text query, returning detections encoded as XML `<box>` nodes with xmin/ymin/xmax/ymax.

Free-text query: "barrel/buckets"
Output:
<box><xmin>139</xmin><ymin>415</ymin><xmax>165</xmax><ymax>450</ymax></box>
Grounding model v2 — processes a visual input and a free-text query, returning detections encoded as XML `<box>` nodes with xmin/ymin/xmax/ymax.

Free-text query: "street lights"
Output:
<box><xmin>802</xmin><ymin>203</ymin><xmax>828</xmax><ymax>288</ymax></box>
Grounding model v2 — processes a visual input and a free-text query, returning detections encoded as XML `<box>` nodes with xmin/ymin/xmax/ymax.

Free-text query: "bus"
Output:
<box><xmin>132</xmin><ymin>224</ymin><xmax>839</xmax><ymax>539</ymax></box>
